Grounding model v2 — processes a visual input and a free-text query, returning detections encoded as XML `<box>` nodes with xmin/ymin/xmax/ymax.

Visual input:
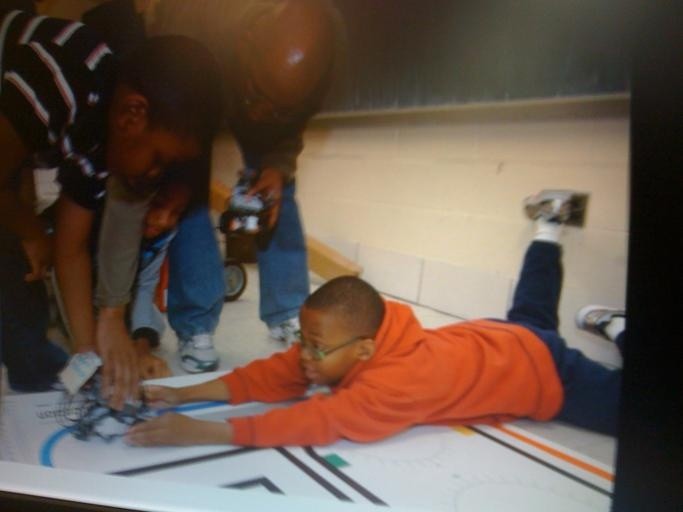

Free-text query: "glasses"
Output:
<box><xmin>293</xmin><ymin>330</ymin><xmax>362</xmax><ymax>362</ymax></box>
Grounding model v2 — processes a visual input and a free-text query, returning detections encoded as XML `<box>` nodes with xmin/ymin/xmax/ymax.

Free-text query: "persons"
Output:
<box><xmin>120</xmin><ymin>185</ymin><xmax>628</xmax><ymax>451</ymax></box>
<box><xmin>125</xmin><ymin>155</ymin><xmax>201</xmax><ymax>380</ymax></box>
<box><xmin>88</xmin><ymin>2</ymin><xmax>338</xmax><ymax>414</ymax></box>
<box><xmin>0</xmin><ymin>3</ymin><xmax>221</xmax><ymax>393</ymax></box>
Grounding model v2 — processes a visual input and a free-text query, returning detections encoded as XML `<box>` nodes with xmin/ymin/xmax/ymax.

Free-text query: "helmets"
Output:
<box><xmin>227</xmin><ymin>1</ymin><xmax>339</xmax><ymax>126</ymax></box>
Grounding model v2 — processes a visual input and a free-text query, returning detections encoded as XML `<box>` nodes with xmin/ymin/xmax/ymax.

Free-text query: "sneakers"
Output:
<box><xmin>7</xmin><ymin>359</ymin><xmax>96</xmax><ymax>393</ymax></box>
<box><xmin>176</xmin><ymin>318</ymin><xmax>221</xmax><ymax>375</ymax></box>
<box><xmin>575</xmin><ymin>304</ymin><xmax>627</xmax><ymax>344</ymax></box>
<box><xmin>267</xmin><ymin>313</ymin><xmax>342</xmax><ymax>349</ymax></box>
<box><xmin>522</xmin><ymin>188</ymin><xmax>592</xmax><ymax>229</ymax></box>
